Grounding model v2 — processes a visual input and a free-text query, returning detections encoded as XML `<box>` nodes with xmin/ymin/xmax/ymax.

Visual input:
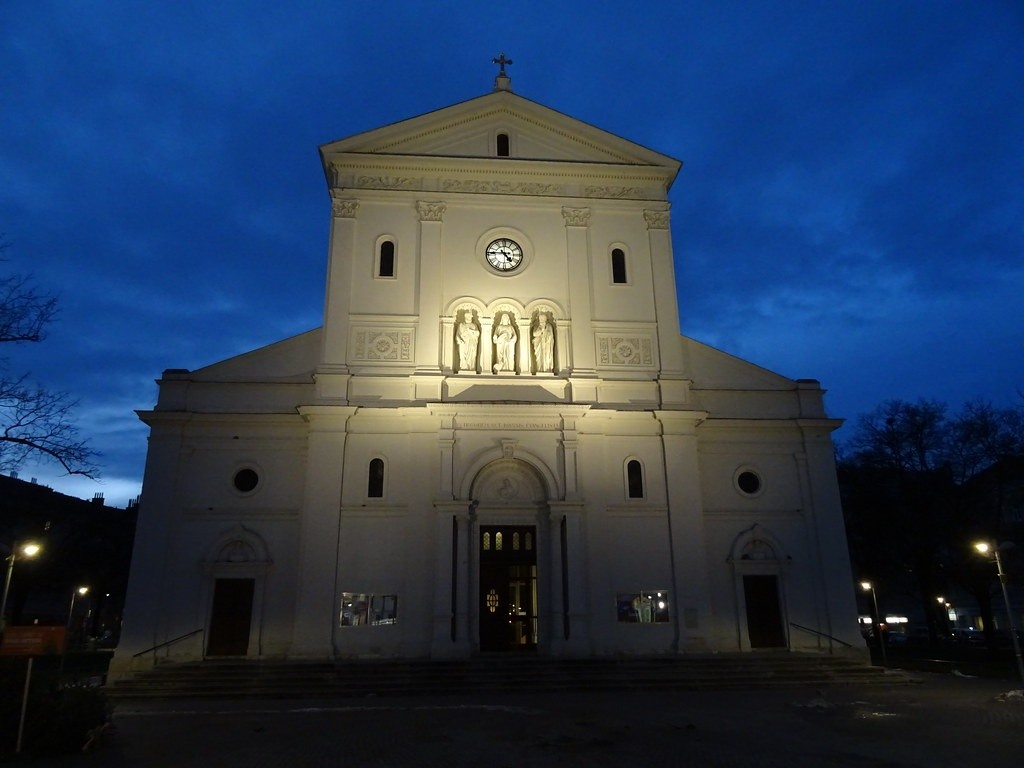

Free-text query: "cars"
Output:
<box><xmin>911</xmin><ymin>624</ymin><xmax>1024</xmax><ymax>647</ymax></box>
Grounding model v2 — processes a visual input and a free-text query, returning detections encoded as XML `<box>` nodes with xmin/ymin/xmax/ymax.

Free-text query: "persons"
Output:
<box><xmin>455</xmin><ymin>312</ymin><xmax>481</xmax><ymax>370</ymax></box>
<box><xmin>531</xmin><ymin>314</ymin><xmax>554</xmax><ymax>372</ymax></box>
<box><xmin>493</xmin><ymin>313</ymin><xmax>517</xmax><ymax>371</ymax></box>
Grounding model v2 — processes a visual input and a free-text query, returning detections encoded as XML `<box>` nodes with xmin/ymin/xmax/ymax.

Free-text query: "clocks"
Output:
<box><xmin>485</xmin><ymin>238</ymin><xmax>524</xmax><ymax>271</ymax></box>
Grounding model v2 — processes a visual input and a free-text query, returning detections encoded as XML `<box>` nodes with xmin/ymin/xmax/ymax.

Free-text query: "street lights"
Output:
<box><xmin>58</xmin><ymin>580</ymin><xmax>89</xmax><ymax>674</ymax></box>
<box><xmin>860</xmin><ymin>578</ymin><xmax>889</xmax><ymax>665</ymax></box>
<box><xmin>0</xmin><ymin>539</ymin><xmax>44</xmax><ymax>633</ymax></box>
<box><xmin>974</xmin><ymin>539</ymin><xmax>1024</xmax><ymax>677</ymax></box>
